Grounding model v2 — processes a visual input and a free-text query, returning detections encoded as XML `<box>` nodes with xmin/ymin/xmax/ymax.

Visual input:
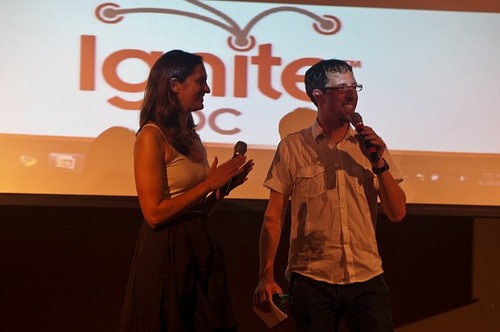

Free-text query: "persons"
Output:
<box><xmin>116</xmin><ymin>48</ymin><xmax>255</xmax><ymax>332</ymax></box>
<box><xmin>251</xmin><ymin>60</ymin><xmax>407</xmax><ymax>332</ymax></box>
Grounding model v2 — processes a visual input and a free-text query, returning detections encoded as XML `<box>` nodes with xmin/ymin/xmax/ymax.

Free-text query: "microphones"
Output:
<box><xmin>225</xmin><ymin>141</ymin><xmax>248</xmax><ymax>195</ymax></box>
<box><xmin>351</xmin><ymin>112</ymin><xmax>379</xmax><ymax>163</ymax></box>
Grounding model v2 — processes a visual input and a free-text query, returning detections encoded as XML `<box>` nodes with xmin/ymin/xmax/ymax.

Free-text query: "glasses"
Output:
<box><xmin>319</xmin><ymin>85</ymin><xmax>362</xmax><ymax>93</ymax></box>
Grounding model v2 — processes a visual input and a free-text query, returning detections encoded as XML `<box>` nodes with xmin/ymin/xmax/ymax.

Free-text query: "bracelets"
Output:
<box><xmin>371</xmin><ymin>157</ymin><xmax>389</xmax><ymax>174</ymax></box>
